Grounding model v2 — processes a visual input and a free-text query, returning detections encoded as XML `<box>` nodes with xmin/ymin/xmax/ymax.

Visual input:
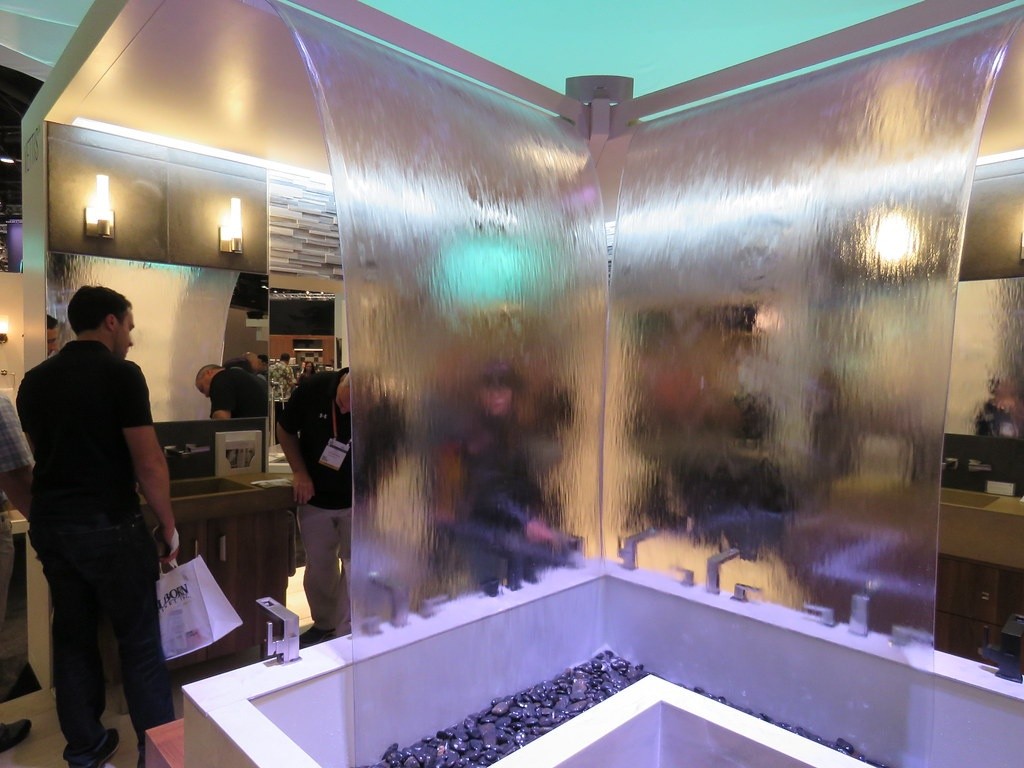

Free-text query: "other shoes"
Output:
<box><xmin>299</xmin><ymin>626</ymin><xmax>336</xmax><ymax>647</ymax></box>
<box><xmin>0</xmin><ymin>719</ymin><xmax>31</xmax><ymax>752</ymax></box>
<box><xmin>72</xmin><ymin>729</ymin><xmax>120</xmax><ymax>768</ymax></box>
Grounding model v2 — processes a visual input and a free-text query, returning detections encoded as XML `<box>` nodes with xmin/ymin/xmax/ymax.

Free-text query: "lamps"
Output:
<box><xmin>84</xmin><ymin>174</ymin><xmax>116</xmax><ymax>239</ymax></box>
<box><xmin>219</xmin><ymin>197</ymin><xmax>244</xmax><ymax>253</ymax></box>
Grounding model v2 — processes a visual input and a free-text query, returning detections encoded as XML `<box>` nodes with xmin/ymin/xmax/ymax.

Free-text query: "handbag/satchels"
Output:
<box><xmin>155</xmin><ymin>558</ymin><xmax>243</xmax><ymax>661</ymax></box>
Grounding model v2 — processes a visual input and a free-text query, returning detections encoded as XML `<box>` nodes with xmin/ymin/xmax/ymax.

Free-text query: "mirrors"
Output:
<box><xmin>45</xmin><ymin>253</ymin><xmax>269</xmax><ymax>424</ymax></box>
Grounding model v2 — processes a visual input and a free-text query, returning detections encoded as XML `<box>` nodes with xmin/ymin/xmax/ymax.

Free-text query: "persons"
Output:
<box><xmin>47</xmin><ymin>314</ymin><xmax>59</xmax><ymax>356</ymax></box>
<box><xmin>16</xmin><ymin>286</ymin><xmax>178</xmax><ymax>768</ymax></box>
<box><xmin>271</xmin><ymin>353</ymin><xmax>292</xmax><ymax>381</ymax></box>
<box><xmin>452</xmin><ymin>362</ymin><xmax>560</xmax><ymax>594</ymax></box>
<box><xmin>976</xmin><ymin>375</ymin><xmax>1024</xmax><ymax>440</ymax></box>
<box><xmin>296</xmin><ymin>357</ymin><xmax>316</xmax><ymax>386</ymax></box>
<box><xmin>0</xmin><ymin>392</ymin><xmax>34</xmax><ymax>752</ymax></box>
<box><xmin>277</xmin><ymin>364</ymin><xmax>402</xmax><ymax>636</ymax></box>
<box><xmin>197</xmin><ymin>355</ymin><xmax>268</xmax><ymax>418</ymax></box>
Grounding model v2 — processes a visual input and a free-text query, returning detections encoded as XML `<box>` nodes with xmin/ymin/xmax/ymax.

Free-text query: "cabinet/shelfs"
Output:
<box><xmin>95</xmin><ymin>504</ymin><xmax>296</xmax><ymax>713</ymax></box>
